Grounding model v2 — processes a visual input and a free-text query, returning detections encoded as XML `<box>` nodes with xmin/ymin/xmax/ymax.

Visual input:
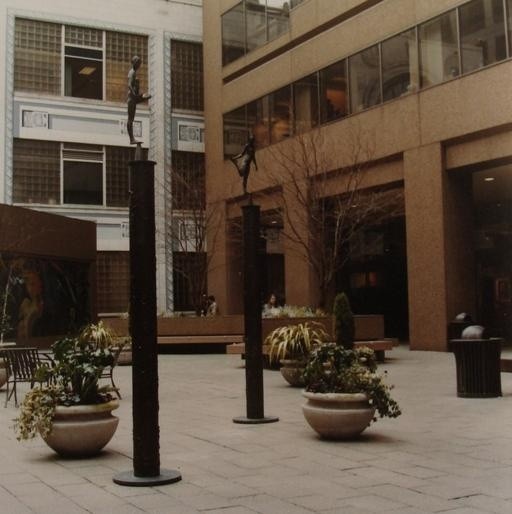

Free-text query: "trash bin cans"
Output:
<box><xmin>449</xmin><ymin>311</ymin><xmax>505</xmax><ymax>398</ymax></box>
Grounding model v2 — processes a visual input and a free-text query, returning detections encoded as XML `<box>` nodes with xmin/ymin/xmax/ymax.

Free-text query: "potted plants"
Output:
<box><xmin>262</xmin><ymin>319</ymin><xmax>401</xmax><ymax>439</ymax></box>
<box><xmin>9</xmin><ymin>321</ymin><xmax>133</xmax><ymax>455</ymax></box>
<box><xmin>0</xmin><ymin>261</ymin><xmax>32</xmax><ymax>388</ymax></box>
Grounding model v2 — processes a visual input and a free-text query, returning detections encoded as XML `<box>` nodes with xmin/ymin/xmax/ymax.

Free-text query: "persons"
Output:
<box><xmin>225</xmin><ymin>132</ymin><xmax>258</xmax><ymax>200</ymax></box>
<box><xmin>196</xmin><ymin>293</ymin><xmax>208</xmax><ymax>316</ymax></box>
<box><xmin>262</xmin><ymin>293</ymin><xmax>282</xmax><ymax>315</ymax></box>
<box><xmin>205</xmin><ymin>295</ymin><xmax>219</xmax><ymax>316</ymax></box>
<box><xmin>127</xmin><ymin>53</ymin><xmax>153</xmax><ymax>145</ymax></box>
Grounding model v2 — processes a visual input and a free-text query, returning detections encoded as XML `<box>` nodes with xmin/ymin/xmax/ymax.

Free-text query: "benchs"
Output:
<box><xmin>157</xmin><ymin>314</ymin><xmax>400</xmax><ymax>370</ymax></box>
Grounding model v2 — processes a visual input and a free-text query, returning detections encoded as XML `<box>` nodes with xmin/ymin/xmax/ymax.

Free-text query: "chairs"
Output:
<box><xmin>1</xmin><ymin>344</ymin><xmax>53</xmax><ymax>409</ymax></box>
